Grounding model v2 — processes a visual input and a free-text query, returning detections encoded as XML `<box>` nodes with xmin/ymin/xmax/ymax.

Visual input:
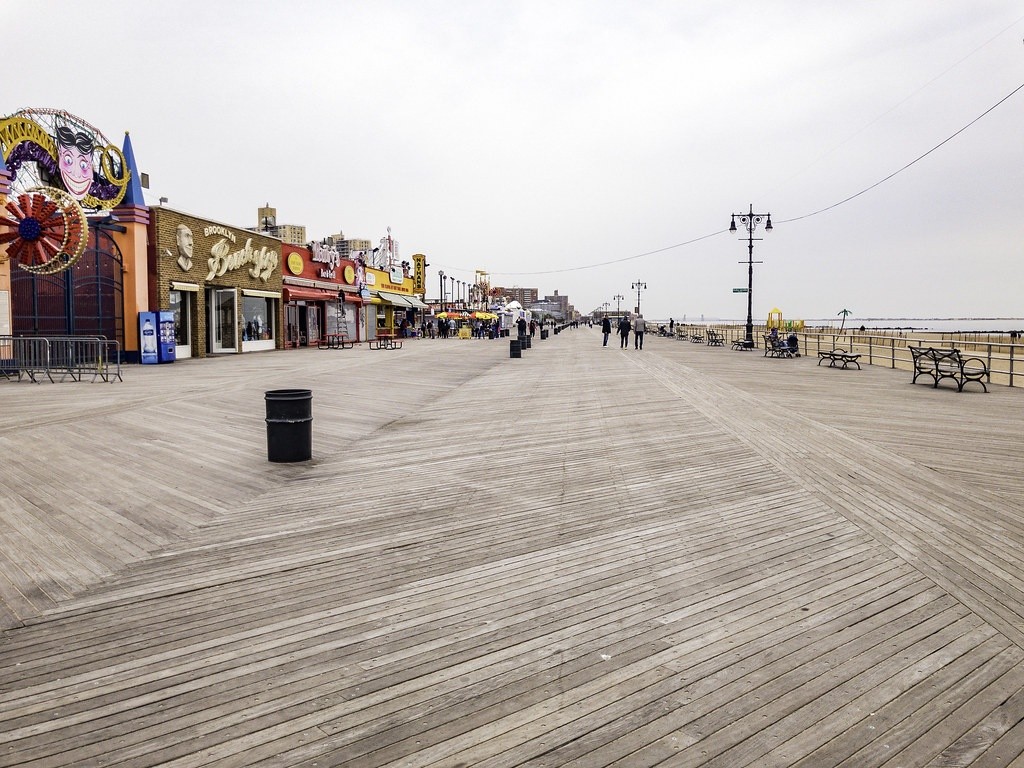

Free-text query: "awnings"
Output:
<box><xmin>378</xmin><ymin>291</ymin><xmax>428</xmax><ymax>308</ymax></box>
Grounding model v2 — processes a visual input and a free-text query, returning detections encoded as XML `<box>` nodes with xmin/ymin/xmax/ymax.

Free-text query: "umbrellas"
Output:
<box><xmin>435</xmin><ymin>310</ymin><xmax>499</xmax><ymax>320</ymax></box>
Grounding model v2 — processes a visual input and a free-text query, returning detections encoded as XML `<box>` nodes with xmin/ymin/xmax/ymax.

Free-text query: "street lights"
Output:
<box><xmin>439</xmin><ymin>270</ymin><xmax>444</xmax><ymax>313</ymax></box>
<box><xmin>462</xmin><ymin>282</ymin><xmax>466</xmax><ymax>299</ymax></box>
<box><xmin>452</xmin><ymin>278</ymin><xmax>455</xmax><ymax>308</ymax></box>
<box><xmin>631</xmin><ymin>279</ymin><xmax>647</xmax><ymax>315</ymax></box>
<box><xmin>603</xmin><ymin>302</ymin><xmax>610</xmax><ymax>315</ymax></box>
<box><xmin>443</xmin><ymin>275</ymin><xmax>447</xmax><ymax>303</ymax></box>
<box><xmin>729</xmin><ymin>204</ymin><xmax>773</xmax><ymax>348</ymax></box>
<box><xmin>457</xmin><ymin>280</ymin><xmax>461</xmax><ymax>309</ymax></box>
<box><xmin>591</xmin><ymin>307</ymin><xmax>603</xmax><ymax>325</ymax></box>
<box><xmin>468</xmin><ymin>284</ymin><xmax>471</xmax><ymax>309</ymax></box>
<box><xmin>614</xmin><ymin>294</ymin><xmax>624</xmax><ymax>326</ymax></box>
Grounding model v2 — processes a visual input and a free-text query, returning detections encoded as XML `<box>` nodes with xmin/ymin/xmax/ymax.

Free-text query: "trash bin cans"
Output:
<box><xmin>554</xmin><ymin>325</ymin><xmax>569</xmax><ymax>334</ymax></box>
<box><xmin>264</xmin><ymin>389</ymin><xmax>313</xmax><ymax>463</ymax></box>
<box><xmin>510</xmin><ymin>340</ymin><xmax>521</xmax><ymax>358</ymax></box>
<box><xmin>541</xmin><ymin>329</ymin><xmax>548</xmax><ymax>339</ymax></box>
<box><xmin>518</xmin><ymin>335</ymin><xmax>532</xmax><ymax>350</ymax></box>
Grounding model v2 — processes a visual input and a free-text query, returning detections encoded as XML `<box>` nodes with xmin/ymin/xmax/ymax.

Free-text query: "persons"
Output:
<box><xmin>176</xmin><ymin>224</ymin><xmax>193</xmax><ymax>271</ymax></box>
<box><xmin>400</xmin><ymin>319</ymin><xmax>410</xmax><ymax>338</ymax></box>
<box><xmin>338</xmin><ymin>289</ymin><xmax>346</xmax><ymax>316</ymax></box>
<box><xmin>616</xmin><ymin>316</ymin><xmax>631</xmax><ymax>350</ymax></box>
<box><xmin>252</xmin><ymin>314</ymin><xmax>262</xmax><ymax>333</ymax></box>
<box><xmin>768</xmin><ymin>328</ymin><xmax>801</xmax><ymax>357</ymax></box>
<box><xmin>1010</xmin><ymin>330</ymin><xmax>1021</xmax><ymax>341</ymax></box>
<box><xmin>569</xmin><ymin>319</ymin><xmax>593</xmax><ymax>329</ymax></box>
<box><xmin>548</xmin><ymin>321</ymin><xmax>556</xmax><ymax>328</ymax></box>
<box><xmin>516</xmin><ymin>316</ymin><xmax>543</xmax><ymax>338</ymax></box>
<box><xmin>633</xmin><ymin>313</ymin><xmax>646</xmax><ymax>350</ymax></box>
<box><xmin>602</xmin><ymin>314</ymin><xmax>612</xmax><ymax>346</ymax></box>
<box><xmin>659</xmin><ymin>318</ymin><xmax>680</xmax><ymax>337</ymax></box>
<box><xmin>471</xmin><ymin>317</ymin><xmax>500</xmax><ymax>339</ymax></box>
<box><xmin>421</xmin><ymin>318</ymin><xmax>456</xmax><ymax>339</ymax></box>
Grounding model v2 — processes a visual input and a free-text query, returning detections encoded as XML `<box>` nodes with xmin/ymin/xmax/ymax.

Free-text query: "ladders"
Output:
<box><xmin>337</xmin><ymin>297</ymin><xmax>350</xmax><ymax>346</ymax></box>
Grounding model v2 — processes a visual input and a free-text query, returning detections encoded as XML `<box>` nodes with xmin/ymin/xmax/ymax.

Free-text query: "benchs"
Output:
<box><xmin>907</xmin><ymin>345</ymin><xmax>990</xmax><ymax>393</ymax></box>
<box><xmin>761</xmin><ymin>335</ymin><xmax>795</xmax><ymax>358</ymax></box>
<box><xmin>732</xmin><ymin>338</ymin><xmax>754</xmax><ymax>350</ymax></box>
<box><xmin>676</xmin><ymin>331</ymin><xmax>705</xmax><ymax>343</ymax></box>
<box><xmin>309</xmin><ymin>339</ymin><xmax>356</xmax><ymax>349</ymax></box>
<box><xmin>818</xmin><ymin>348</ymin><xmax>861</xmax><ymax>370</ymax></box>
<box><xmin>706</xmin><ymin>330</ymin><xmax>725</xmax><ymax>346</ymax></box>
<box><xmin>369</xmin><ymin>341</ymin><xmax>404</xmax><ymax>350</ymax></box>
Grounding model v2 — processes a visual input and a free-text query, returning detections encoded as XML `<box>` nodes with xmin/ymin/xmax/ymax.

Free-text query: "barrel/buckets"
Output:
<box><xmin>266</xmin><ymin>389</ymin><xmax>312</xmax><ymax>462</ymax></box>
<box><xmin>554</xmin><ymin>324</ymin><xmax>569</xmax><ymax>334</ymax></box>
<box><xmin>510</xmin><ymin>335</ymin><xmax>531</xmax><ymax>359</ymax></box>
<box><xmin>541</xmin><ymin>329</ymin><xmax>548</xmax><ymax>340</ymax></box>
<box><xmin>489</xmin><ymin>329</ymin><xmax>510</xmax><ymax>339</ymax></box>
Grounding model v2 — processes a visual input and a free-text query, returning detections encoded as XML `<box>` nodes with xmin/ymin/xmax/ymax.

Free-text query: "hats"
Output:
<box><xmin>624</xmin><ymin>316</ymin><xmax>627</xmax><ymax>320</ymax></box>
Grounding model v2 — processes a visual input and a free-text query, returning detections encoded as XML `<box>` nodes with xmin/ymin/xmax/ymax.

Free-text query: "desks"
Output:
<box><xmin>323</xmin><ymin>333</ymin><xmax>348</xmax><ymax>349</ymax></box>
<box><xmin>375</xmin><ymin>334</ymin><xmax>397</xmax><ymax>350</ymax></box>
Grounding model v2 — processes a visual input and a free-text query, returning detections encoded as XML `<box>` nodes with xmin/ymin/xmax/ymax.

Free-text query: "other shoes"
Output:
<box><xmin>625</xmin><ymin>348</ymin><xmax>627</xmax><ymax>350</ymax></box>
<box><xmin>635</xmin><ymin>347</ymin><xmax>637</xmax><ymax>349</ymax></box>
<box><xmin>604</xmin><ymin>346</ymin><xmax>608</xmax><ymax>348</ymax></box>
<box><xmin>640</xmin><ymin>347</ymin><xmax>642</xmax><ymax>349</ymax></box>
